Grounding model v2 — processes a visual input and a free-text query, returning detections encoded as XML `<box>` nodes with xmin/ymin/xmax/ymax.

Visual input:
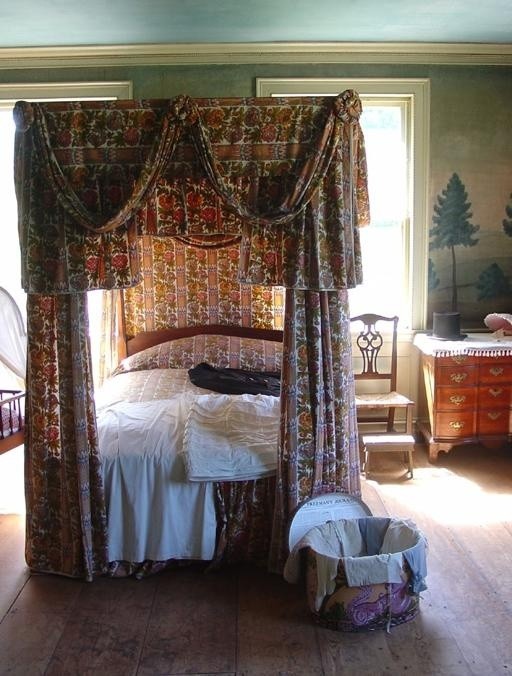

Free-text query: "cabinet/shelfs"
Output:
<box><xmin>415</xmin><ymin>333</ymin><xmax>512</xmax><ymax>462</ymax></box>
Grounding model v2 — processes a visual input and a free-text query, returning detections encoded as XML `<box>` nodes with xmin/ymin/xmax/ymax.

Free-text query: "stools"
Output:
<box><xmin>361</xmin><ymin>433</ymin><xmax>416</xmax><ymax>478</ymax></box>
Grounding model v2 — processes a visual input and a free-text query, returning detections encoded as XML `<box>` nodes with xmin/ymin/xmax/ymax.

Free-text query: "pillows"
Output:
<box><xmin>111</xmin><ymin>336</ymin><xmax>283</xmax><ymax>375</ymax></box>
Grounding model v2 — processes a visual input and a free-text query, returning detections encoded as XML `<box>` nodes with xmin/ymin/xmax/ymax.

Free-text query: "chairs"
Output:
<box><xmin>349</xmin><ymin>313</ymin><xmax>416</xmax><ymax>459</ymax></box>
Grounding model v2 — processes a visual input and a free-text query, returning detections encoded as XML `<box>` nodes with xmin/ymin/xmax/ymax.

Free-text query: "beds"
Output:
<box><xmin>0</xmin><ymin>285</ymin><xmax>26</xmax><ymax>455</ymax></box>
<box><xmin>14</xmin><ymin>88</ymin><xmax>371</xmax><ymax>582</ymax></box>
